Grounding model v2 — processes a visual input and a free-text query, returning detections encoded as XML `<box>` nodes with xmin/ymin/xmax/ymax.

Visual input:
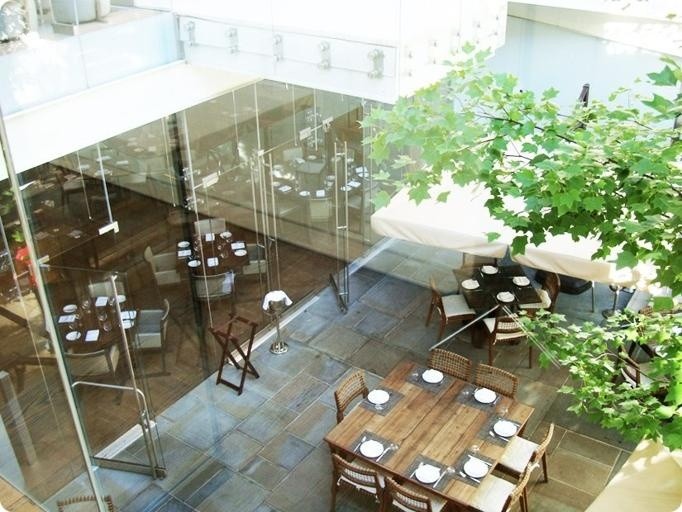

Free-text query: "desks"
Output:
<box><xmin>167</xmin><ymin>209</ymin><xmax>197</xmax><ymax>242</ymax></box>
<box><xmin>324</xmin><ymin>356</ymin><xmax>535</xmax><ymax>511</ymax></box>
<box><xmin>176</xmin><ymin>234</ymin><xmax>252</xmax><ymax>300</ymax></box>
<box><xmin>452</xmin><ymin>263</ymin><xmax>543</xmax><ymax>349</ymax></box>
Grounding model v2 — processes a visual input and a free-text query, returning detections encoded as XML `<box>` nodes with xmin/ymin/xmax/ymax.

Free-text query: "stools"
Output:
<box><xmin>534</xmin><ymin>269</ymin><xmax>598</xmax><ymax>313</ymax></box>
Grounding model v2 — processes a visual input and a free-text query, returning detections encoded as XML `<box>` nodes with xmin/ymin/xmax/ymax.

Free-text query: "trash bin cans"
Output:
<box><xmin>617</xmin><ymin>286</ymin><xmax>636</xmax><ymax>314</ymax></box>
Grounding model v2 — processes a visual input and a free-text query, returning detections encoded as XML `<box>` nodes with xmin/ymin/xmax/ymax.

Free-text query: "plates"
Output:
<box><xmin>265</xmin><ymin>151</ymin><xmax>372</xmax><ymax>197</ymax></box>
<box><xmin>55</xmin><ymin>230</ymin><xmax>249</xmax><ymax>347</ymax></box>
<box><xmin>354</xmin><ymin>369</ymin><xmax>517</xmax><ymax>483</ymax></box>
<box><xmin>459</xmin><ymin>265</ymin><xmax>531</xmax><ymax>303</ymax></box>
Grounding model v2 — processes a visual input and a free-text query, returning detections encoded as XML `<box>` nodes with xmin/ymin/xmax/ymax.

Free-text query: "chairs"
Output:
<box><xmin>495</xmin><ymin>421</ymin><xmax>556</xmax><ymax>511</ymax></box>
<box><xmin>474</xmin><ymin>360</ymin><xmax>518</xmax><ymax>399</ymax></box>
<box><xmin>334</xmin><ymin>367</ymin><xmax>370</xmax><ymax>461</ymax></box>
<box><xmin>383</xmin><ymin>475</ymin><xmax>449</xmax><ymax>512</ymax></box>
<box><xmin>481</xmin><ymin>297</ymin><xmax>538</xmax><ymax>370</ymax></box>
<box><xmin>424</xmin><ymin>274</ymin><xmax>476</xmax><ymax>351</ymax></box>
<box><xmin>328</xmin><ymin>454</ymin><xmax>395</xmax><ymax>512</ymax></box>
<box><xmin>143</xmin><ymin>245</ymin><xmax>188</xmax><ymax>287</ymax></box>
<box><xmin>191</xmin><ymin>267</ymin><xmax>238</xmax><ymax>326</ymax></box>
<box><xmin>242</xmin><ymin>235</ymin><xmax>276</xmax><ymax>282</ymax></box>
<box><xmin>0</xmin><ymin>161</ymin><xmax>171</xmax><ymax>405</ymax></box>
<box><xmin>527</xmin><ymin>270</ymin><xmax>560</xmax><ymax>321</ymax></box>
<box><xmin>457</xmin><ymin>252</ymin><xmax>498</xmax><ymax>295</ymax></box>
<box><xmin>424</xmin><ymin>346</ymin><xmax>472</xmax><ymax>382</ymax></box>
<box><xmin>193</xmin><ymin>216</ymin><xmax>227</xmax><ymax>235</ymax></box>
<box><xmin>449</xmin><ymin>460</ymin><xmax>536</xmax><ymax>511</ymax></box>
